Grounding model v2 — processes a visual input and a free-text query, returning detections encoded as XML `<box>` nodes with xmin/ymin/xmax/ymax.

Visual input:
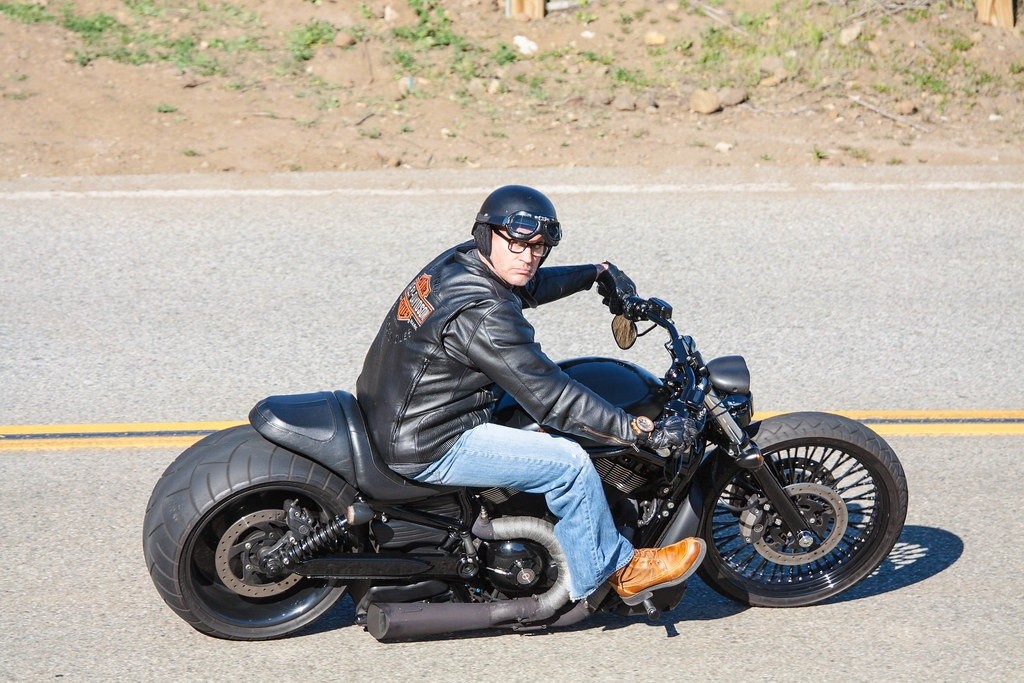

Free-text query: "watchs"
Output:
<box><xmin>629</xmin><ymin>413</ymin><xmax>655</xmax><ymax>455</ymax></box>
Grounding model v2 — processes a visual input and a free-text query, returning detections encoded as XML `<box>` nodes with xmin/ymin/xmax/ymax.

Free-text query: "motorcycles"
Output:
<box><xmin>141</xmin><ymin>261</ymin><xmax>911</xmax><ymax>644</ymax></box>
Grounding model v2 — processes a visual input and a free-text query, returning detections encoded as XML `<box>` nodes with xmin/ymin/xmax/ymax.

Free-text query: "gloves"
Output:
<box><xmin>638</xmin><ymin>416</ymin><xmax>698</xmax><ymax>461</ymax></box>
<box><xmin>595</xmin><ymin>260</ymin><xmax>636</xmax><ymax>316</ymax></box>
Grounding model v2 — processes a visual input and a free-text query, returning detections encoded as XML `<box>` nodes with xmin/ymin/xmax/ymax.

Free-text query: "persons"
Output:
<box><xmin>354</xmin><ymin>185</ymin><xmax>708</xmax><ymax>609</ymax></box>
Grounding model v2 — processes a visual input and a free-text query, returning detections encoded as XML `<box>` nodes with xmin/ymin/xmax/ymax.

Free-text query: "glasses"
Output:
<box><xmin>491</xmin><ymin>225</ymin><xmax>550</xmax><ymax>258</ymax></box>
<box><xmin>502</xmin><ymin>210</ymin><xmax>562</xmax><ymax>246</ymax></box>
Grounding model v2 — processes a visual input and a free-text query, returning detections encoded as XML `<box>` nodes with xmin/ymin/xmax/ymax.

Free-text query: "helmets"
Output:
<box><xmin>471</xmin><ymin>185</ymin><xmax>563</xmax><ymax>270</ymax></box>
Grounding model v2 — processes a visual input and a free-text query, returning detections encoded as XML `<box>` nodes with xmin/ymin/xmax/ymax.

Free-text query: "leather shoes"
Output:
<box><xmin>608</xmin><ymin>537</ymin><xmax>707</xmax><ymax>607</ymax></box>
